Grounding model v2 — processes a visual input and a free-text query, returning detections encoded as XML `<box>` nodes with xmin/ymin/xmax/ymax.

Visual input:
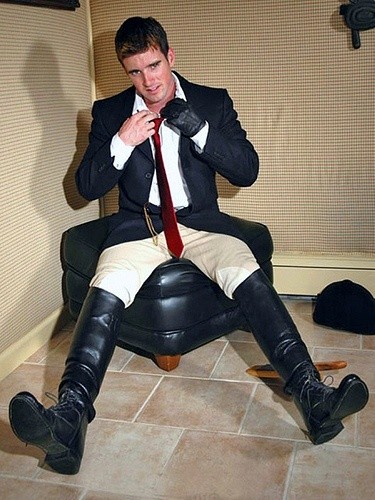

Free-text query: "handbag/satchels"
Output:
<box><xmin>313</xmin><ymin>279</ymin><xmax>375</xmax><ymax>336</ymax></box>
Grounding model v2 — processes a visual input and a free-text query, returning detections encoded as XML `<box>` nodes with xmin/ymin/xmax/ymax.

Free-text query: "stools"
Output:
<box><xmin>63</xmin><ymin>211</ymin><xmax>274</xmax><ymax>371</ymax></box>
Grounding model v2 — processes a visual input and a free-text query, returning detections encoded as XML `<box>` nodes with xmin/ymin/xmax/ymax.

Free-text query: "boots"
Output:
<box><xmin>284</xmin><ymin>363</ymin><xmax>369</xmax><ymax>445</ymax></box>
<box><xmin>9</xmin><ymin>387</ymin><xmax>89</xmax><ymax>475</ymax></box>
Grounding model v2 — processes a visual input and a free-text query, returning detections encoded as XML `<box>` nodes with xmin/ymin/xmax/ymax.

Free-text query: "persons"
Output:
<box><xmin>8</xmin><ymin>16</ymin><xmax>370</xmax><ymax>476</ymax></box>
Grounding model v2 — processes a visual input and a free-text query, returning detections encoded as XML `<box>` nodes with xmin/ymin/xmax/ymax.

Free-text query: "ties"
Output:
<box><xmin>149</xmin><ymin>116</ymin><xmax>184</xmax><ymax>259</ymax></box>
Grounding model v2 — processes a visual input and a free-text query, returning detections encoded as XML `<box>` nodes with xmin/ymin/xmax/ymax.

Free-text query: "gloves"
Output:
<box><xmin>159</xmin><ymin>97</ymin><xmax>206</xmax><ymax>137</ymax></box>
